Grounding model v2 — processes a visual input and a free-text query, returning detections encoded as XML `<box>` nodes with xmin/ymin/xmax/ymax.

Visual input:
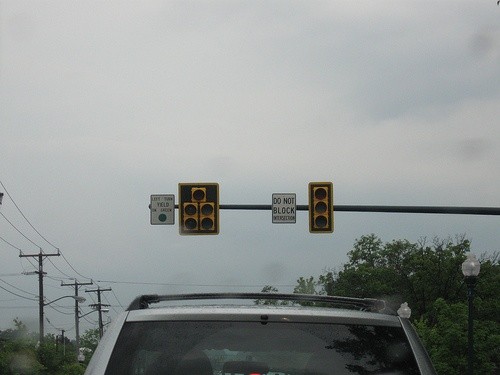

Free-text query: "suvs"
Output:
<box><xmin>83</xmin><ymin>293</ymin><xmax>436</xmax><ymax>375</ymax></box>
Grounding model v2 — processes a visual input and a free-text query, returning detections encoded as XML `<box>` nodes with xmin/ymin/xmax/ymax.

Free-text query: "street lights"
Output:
<box><xmin>461</xmin><ymin>253</ymin><xmax>481</xmax><ymax>375</ymax></box>
<box><xmin>75</xmin><ymin>308</ymin><xmax>110</xmax><ymax>361</ymax></box>
<box><xmin>39</xmin><ymin>294</ymin><xmax>86</xmax><ymax>375</ymax></box>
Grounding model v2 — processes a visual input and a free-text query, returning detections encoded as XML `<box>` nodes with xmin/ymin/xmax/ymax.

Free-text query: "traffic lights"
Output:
<box><xmin>307</xmin><ymin>182</ymin><xmax>335</xmax><ymax>234</ymax></box>
<box><xmin>177</xmin><ymin>182</ymin><xmax>219</xmax><ymax>236</ymax></box>
